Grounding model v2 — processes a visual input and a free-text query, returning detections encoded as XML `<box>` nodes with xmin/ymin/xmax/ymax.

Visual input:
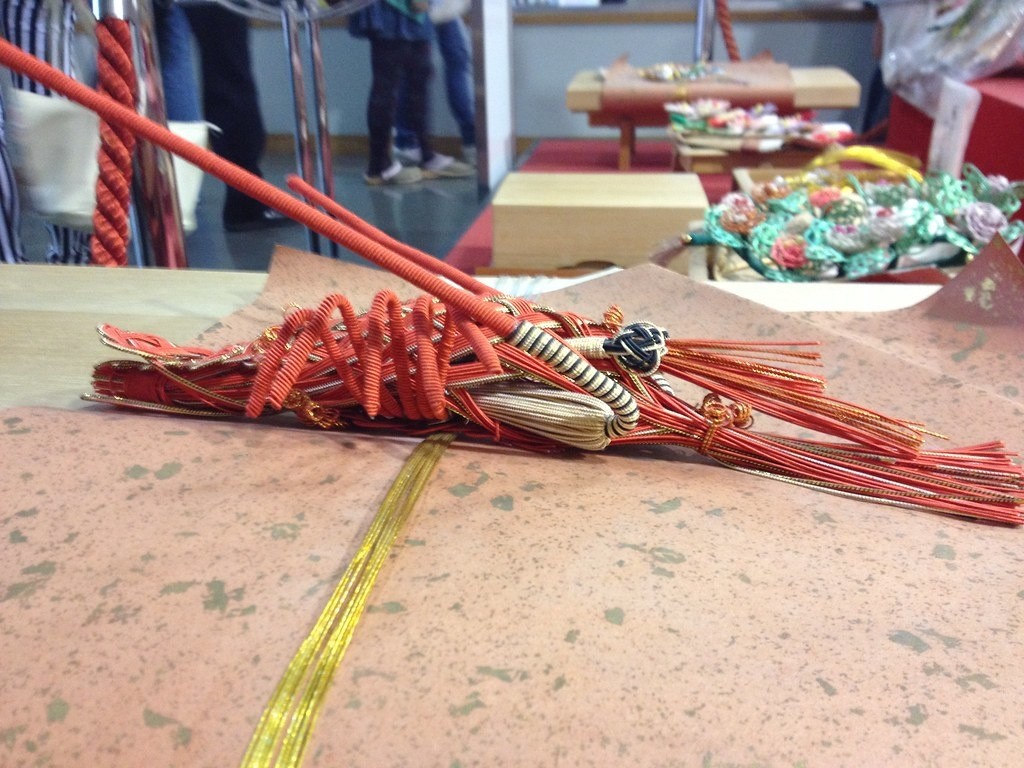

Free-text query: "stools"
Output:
<box><xmin>490</xmin><ymin>172</ymin><xmax>711</xmax><ymax>276</ymax></box>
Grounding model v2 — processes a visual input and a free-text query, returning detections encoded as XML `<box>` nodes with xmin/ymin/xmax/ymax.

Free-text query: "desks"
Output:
<box><xmin>0</xmin><ymin>262</ymin><xmax>1024</xmax><ymax>768</ymax></box>
<box><xmin>568</xmin><ymin>66</ymin><xmax>862</xmax><ymax>169</ymax></box>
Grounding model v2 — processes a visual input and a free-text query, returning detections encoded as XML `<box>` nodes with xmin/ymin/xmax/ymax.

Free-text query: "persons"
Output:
<box><xmin>150</xmin><ymin>0</ymin><xmax>302</xmax><ymax>232</ymax></box>
<box><xmin>347</xmin><ymin>0</ymin><xmax>480</xmax><ymax>184</ymax></box>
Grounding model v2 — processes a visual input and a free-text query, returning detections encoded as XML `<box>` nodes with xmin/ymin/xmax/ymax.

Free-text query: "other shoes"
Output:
<box><xmin>364</xmin><ymin>162</ymin><xmax>421</xmax><ymax>185</ymax></box>
<box><xmin>425</xmin><ymin>155</ymin><xmax>476</xmax><ymax>178</ymax></box>
<box><xmin>221</xmin><ymin>202</ymin><xmax>301</xmax><ymax>232</ymax></box>
